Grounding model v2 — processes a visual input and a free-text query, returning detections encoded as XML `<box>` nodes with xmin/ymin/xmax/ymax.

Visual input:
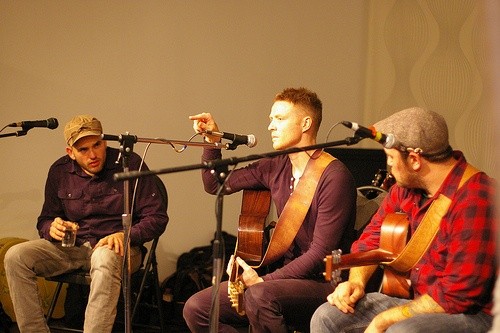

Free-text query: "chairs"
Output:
<box><xmin>248</xmin><ymin>186</ymin><xmax>389</xmax><ymax>333</ymax></box>
<box><xmin>45</xmin><ymin>235</ymin><xmax>166</xmax><ymax>333</ymax></box>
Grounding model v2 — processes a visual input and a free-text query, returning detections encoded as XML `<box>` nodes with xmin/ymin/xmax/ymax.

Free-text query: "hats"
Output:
<box><xmin>372</xmin><ymin>107</ymin><xmax>449</xmax><ymax>152</ymax></box>
<box><xmin>64</xmin><ymin>115</ymin><xmax>103</xmax><ymax>147</ymax></box>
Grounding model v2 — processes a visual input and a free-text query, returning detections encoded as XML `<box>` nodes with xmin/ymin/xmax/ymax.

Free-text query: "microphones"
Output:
<box><xmin>8</xmin><ymin>118</ymin><xmax>59</xmax><ymax>130</ymax></box>
<box><xmin>342</xmin><ymin>121</ymin><xmax>396</xmax><ymax>149</ymax></box>
<box><xmin>205</xmin><ymin>130</ymin><xmax>257</xmax><ymax>148</ymax></box>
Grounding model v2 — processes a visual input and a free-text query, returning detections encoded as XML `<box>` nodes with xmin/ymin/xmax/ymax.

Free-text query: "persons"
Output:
<box><xmin>183</xmin><ymin>86</ymin><xmax>356</xmax><ymax>333</ymax></box>
<box><xmin>309</xmin><ymin>107</ymin><xmax>500</xmax><ymax>333</ymax></box>
<box><xmin>4</xmin><ymin>115</ymin><xmax>168</xmax><ymax>333</ymax></box>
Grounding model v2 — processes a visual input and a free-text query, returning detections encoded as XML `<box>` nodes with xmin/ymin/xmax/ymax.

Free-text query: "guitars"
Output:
<box><xmin>320</xmin><ymin>211</ymin><xmax>413</xmax><ymax>299</ymax></box>
<box><xmin>226</xmin><ymin>186</ymin><xmax>273</xmax><ymax>317</ymax></box>
